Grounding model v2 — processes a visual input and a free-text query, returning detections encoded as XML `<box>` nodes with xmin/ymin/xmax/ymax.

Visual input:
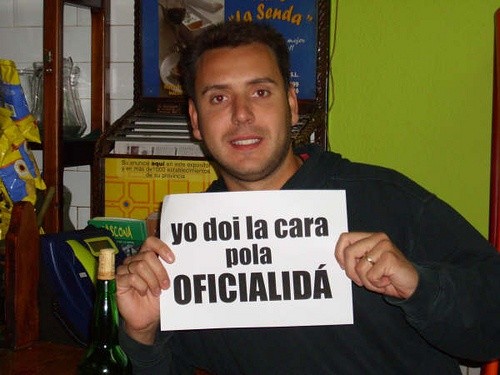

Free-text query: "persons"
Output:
<box><xmin>114</xmin><ymin>19</ymin><xmax>500</xmax><ymax>375</ymax></box>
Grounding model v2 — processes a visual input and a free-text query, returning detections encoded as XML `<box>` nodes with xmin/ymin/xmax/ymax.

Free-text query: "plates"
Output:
<box><xmin>160</xmin><ymin>53</ymin><xmax>184</xmax><ymax>94</ymax></box>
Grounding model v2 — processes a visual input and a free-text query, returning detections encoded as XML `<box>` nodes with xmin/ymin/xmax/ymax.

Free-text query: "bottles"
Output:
<box><xmin>78</xmin><ymin>249</ymin><xmax>132</xmax><ymax>375</ymax></box>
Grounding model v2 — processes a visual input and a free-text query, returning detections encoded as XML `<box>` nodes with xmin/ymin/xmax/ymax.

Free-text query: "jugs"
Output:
<box><xmin>30</xmin><ymin>57</ymin><xmax>87</xmax><ymax>141</ymax></box>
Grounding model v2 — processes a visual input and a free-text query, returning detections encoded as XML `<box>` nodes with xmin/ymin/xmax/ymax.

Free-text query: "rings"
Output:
<box><xmin>364</xmin><ymin>254</ymin><xmax>375</xmax><ymax>265</ymax></box>
<box><xmin>126</xmin><ymin>262</ymin><xmax>134</xmax><ymax>273</ymax></box>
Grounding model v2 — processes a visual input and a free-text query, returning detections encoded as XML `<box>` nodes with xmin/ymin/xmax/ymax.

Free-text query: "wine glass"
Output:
<box><xmin>167</xmin><ymin>0</ymin><xmax>188</xmax><ymax>51</ymax></box>
